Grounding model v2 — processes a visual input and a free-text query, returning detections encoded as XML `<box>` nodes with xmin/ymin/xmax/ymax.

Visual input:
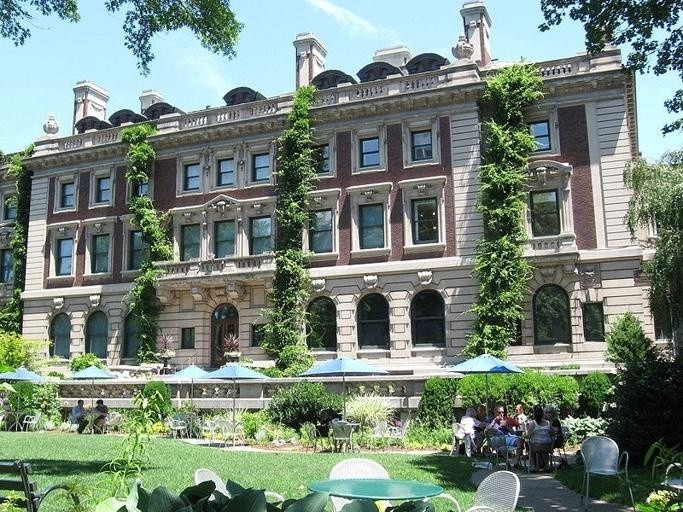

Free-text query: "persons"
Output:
<box><xmin>71</xmin><ymin>400</ymin><xmax>89</xmax><ymax>434</ymax></box>
<box><xmin>523</xmin><ymin>405</ymin><xmax>553</xmax><ymax>472</ymax></box>
<box><xmin>92</xmin><ymin>400</ymin><xmax>108</xmax><ymax>433</ymax></box>
<box><xmin>460</xmin><ymin>407</ymin><xmax>484</xmax><ymax>456</ymax></box>
<box><xmin>543</xmin><ymin>407</ymin><xmax>565</xmax><ymax>449</ymax></box>
<box><xmin>483</xmin><ymin>405</ymin><xmax>524</xmax><ymax>469</ymax></box>
<box><xmin>509</xmin><ymin>404</ymin><xmax>526</xmax><ymax>429</ymax></box>
<box><xmin>475</xmin><ymin>405</ymin><xmax>487</xmax><ymax>431</ymax></box>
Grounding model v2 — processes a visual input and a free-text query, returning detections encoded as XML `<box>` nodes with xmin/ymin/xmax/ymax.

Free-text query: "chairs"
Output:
<box><xmin>163</xmin><ymin>412</ymin><xmax>247</xmax><ymax>448</ymax></box>
<box><xmin>444</xmin><ymin>410</ymin><xmax>571</xmax><ymax>473</ymax></box>
<box><xmin>419</xmin><ymin>469</ymin><xmax>521</xmax><ymax>512</ymax></box>
<box><xmin>65</xmin><ymin>410</ymin><xmax>122</xmax><ymax>436</ymax></box>
<box><xmin>325</xmin><ymin>457</ymin><xmax>394</xmax><ymax>511</ymax></box>
<box><xmin>0</xmin><ymin>408</ymin><xmax>42</xmax><ymax>433</ymax></box>
<box><xmin>579</xmin><ymin>435</ymin><xmax>637</xmax><ymax>511</ymax></box>
<box><xmin>192</xmin><ymin>467</ymin><xmax>286</xmax><ymax>505</ymax></box>
<box><xmin>0</xmin><ymin>458</ymin><xmax>83</xmax><ymax>512</ymax></box>
<box><xmin>658</xmin><ymin>460</ymin><xmax>682</xmax><ymax>497</ymax></box>
<box><xmin>297</xmin><ymin>416</ymin><xmax>415</xmax><ymax>453</ymax></box>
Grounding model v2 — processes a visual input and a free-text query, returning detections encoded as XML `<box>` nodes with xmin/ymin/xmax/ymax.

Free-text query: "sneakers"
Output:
<box><xmin>514</xmin><ymin>463</ymin><xmax>523</xmax><ymax>470</ymax></box>
<box><xmin>505</xmin><ymin>461</ymin><xmax>511</xmax><ymax>469</ymax></box>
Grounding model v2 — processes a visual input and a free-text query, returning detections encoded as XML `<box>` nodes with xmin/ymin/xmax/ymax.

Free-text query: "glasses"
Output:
<box><xmin>499</xmin><ymin>411</ymin><xmax>504</xmax><ymax>414</ymax></box>
<box><xmin>544</xmin><ymin>411</ymin><xmax>552</xmax><ymax>414</ymax></box>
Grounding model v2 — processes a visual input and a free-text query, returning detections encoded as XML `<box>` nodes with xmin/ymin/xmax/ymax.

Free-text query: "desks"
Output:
<box><xmin>306</xmin><ymin>477</ymin><xmax>444</xmax><ymax>501</ymax></box>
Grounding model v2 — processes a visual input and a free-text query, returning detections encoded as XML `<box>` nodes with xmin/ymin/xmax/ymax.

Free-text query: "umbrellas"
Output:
<box><xmin>0</xmin><ymin>363</ymin><xmax>48</xmax><ymax>431</ymax></box>
<box><xmin>68</xmin><ymin>365</ymin><xmax>117</xmax><ymax>419</ymax></box>
<box><xmin>167</xmin><ymin>364</ymin><xmax>209</xmax><ymax>439</ymax></box>
<box><xmin>295</xmin><ymin>355</ymin><xmax>386</xmax><ymax>418</ymax></box>
<box><xmin>444</xmin><ymin>353</ymin><xmax>516</xmax><ymax>413</ymax></box>
<box><xmin>198</xmin><ymin>365</ymin><xmax>269</xmax><ymax>448</ymax></box>
